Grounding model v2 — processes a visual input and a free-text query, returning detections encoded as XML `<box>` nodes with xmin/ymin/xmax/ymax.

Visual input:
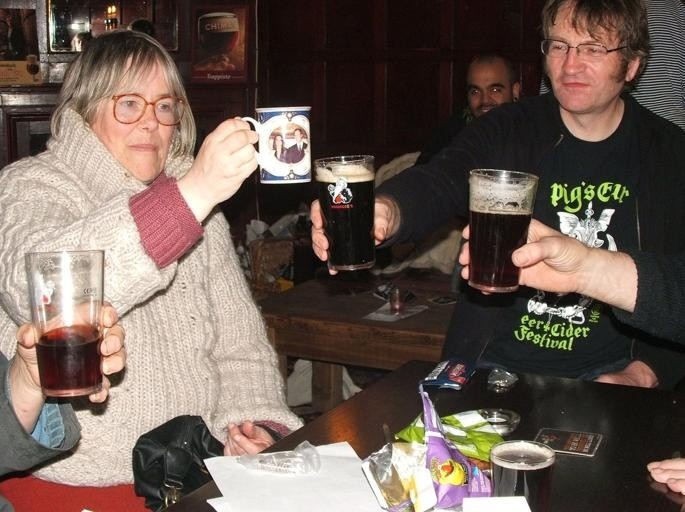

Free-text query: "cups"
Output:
<box><xmin>471</xmin><ymin>171</ymin><xmax>538</xmax><ymax>296</ymax></box>
<box><xmin>315</xmin><ymin>154</ymin><xmax>378</xmax><ymax>275</ymax></box>
<box><xmin>489</xmin><ymin>441</ymin><xmax>556</xmax><ymax>511</ymax></box>
<box><xmin>242</xmin><ymin>107</ymin><xmax>314</xmax><ymax>185</ymax></box>
<box><xmin>26</xmin><ymin>251</ymin><xmax>112</xmax><ymax>397</ymax></box>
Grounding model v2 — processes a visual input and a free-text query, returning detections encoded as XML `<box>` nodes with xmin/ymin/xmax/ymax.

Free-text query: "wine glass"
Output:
<box><xmin>190</xmin><ymin>12</ymin><xmax>249</xmax><ymax>82</ymax></box>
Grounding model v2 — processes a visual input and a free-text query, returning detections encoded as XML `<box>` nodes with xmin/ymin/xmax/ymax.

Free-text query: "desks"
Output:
<box><xmin>258</xmin><ymin>261</ymin><xmax>456</xmax><ymax>413</ymax></box>
<box><xmin>165</xmin><ymin>359</ymin><xmax>685</xmax><ymax>512</ymax></box>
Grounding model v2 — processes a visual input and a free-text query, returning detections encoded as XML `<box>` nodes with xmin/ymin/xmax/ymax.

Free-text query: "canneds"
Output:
<box><xmin>391</xmin><ymin>286</ymin><xmax>405</xmax><ymax>315</ymax></box>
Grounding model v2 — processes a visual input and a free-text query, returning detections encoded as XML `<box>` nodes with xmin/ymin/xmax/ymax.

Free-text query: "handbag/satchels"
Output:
<box><xmin>132</xmin><ymin>414</ymin><xmax>226</xmax><ymax>511</ymax></box>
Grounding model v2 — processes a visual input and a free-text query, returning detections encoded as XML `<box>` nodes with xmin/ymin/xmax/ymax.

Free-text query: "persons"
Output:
<box><xmin>458</xmin><ymin>215</ymin><xmax>685</xmax><ymax>499</ymax></box>
<box><xmin>271</xmin><ymin>134</ymin><xmax>286</xmax><ymax>161</ymax></box>
<box><xmin>181</xmin><ymin>56</ymin><xmax>235</xmax><ymax>72</ymax></box>
<box><xmin>0</xmin><ymin>31</ymin><xmax>305</xmax><ymax>487</ymax></box>
<box><xmin>0</xmin><ymin>299</ymin><xmax>128</xmax><ymax>512</ymax></box>
<box><xmin>310</xmin><ymin>0</ymin><xmax>684</xmax><ymax>388</ymax></box>
<box><xmin>539</xmin><ymin>0</ymin><xmax>685</xmax><ymax>130</ymax></box>
<box><xmin>127</xmin><ymin>18</ymin><xmax>161</xmax><ymax>45</ymax></box>
<box><xmin>70</xmin><ymin>31</ymin><xmax>93</xmax><ymax>52</ymax></box>
<box><xmin>284</xmin><ymin>128</ymin><xmax>307</xmax><ymax>162</ymax></box>
<box><xmin>413</xmin><ymin>40</ymin><xmax>520</xmax><ymax>164</ymax></box>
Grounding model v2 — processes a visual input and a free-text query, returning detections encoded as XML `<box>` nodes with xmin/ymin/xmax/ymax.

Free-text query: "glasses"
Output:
<box><xmin>106</xmin><ymin>94</ymin><xmax>185</xmax><ymax>126</ymax></box>
<box><xmin>541</xmin><ymin>39</ymin><xmax>627</xmax><ymax>61</ymax></box>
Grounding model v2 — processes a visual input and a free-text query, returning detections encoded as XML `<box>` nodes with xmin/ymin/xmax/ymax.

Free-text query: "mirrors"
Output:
<box><xmin>35</xmin><ymin>1</ymin><xmax>189</xmax><ymax>60</ymax></box>
